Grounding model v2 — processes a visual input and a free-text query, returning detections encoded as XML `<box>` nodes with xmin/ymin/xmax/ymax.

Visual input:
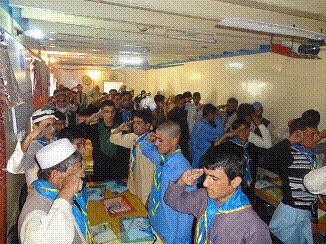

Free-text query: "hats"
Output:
<box><xmin>32</xmin><ymin>108</ymin><xmax>59</xmax><ymax>123</ymax></box>
<box><xmin>36</xmin><ymin>138</ymin><xmax>77</xmax><ymax>169</ymax></box>
<box><xmin>252</xmin><ymin>102</ymin><xmax>262</xmax><ymax>109</ymax></box>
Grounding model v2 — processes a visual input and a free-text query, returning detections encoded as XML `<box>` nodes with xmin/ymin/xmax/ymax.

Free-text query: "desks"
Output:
<box><xmin>254</xmin><ymin>174</ymin><xmax>326</xmax><ymax>235</ymax></box>
<box><xmin>84</xmin><ymin>180</ymin><xmax>163</xmax><ymax>243</ymax></box>
<box><xmin>77</xmin><ymin>138</ymin><xmax>94</xmax><ymax>172</ymax></box>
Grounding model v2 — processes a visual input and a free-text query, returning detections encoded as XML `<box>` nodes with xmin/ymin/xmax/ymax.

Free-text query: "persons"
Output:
<box><xmin>70</xmin><ymin>100</ymin><xmax>131</xmax><ymax>181</ymax></box>
<box><xmin>17</xmin><ymin>137</ymin><xmax>92</xmax><ymax>244</ymax></box>
<box><xmin>262</xmin><ymin>117</ymin><xmax>321</xmax><ymax>244</ymax></box>
<box><xmin>237</xmin><ymin>101</ymin><xmax>274</xmax><ymax>149</ymax></box>
<box><xmin>109</xmin><ymin>110</ymin><xmax>156</xmax><ymax>211</ymax></box>
<box><xmin>45</xmin><ymin>81</ymin><xmax>241</xmax><ymax>128</ymax></box>
<box><xmin>7</xmin><ymin>109</ymin><xmax>58</xmax><ymax>195</ymax></box>
<box><xmin>139</xmin><ymin>120</ymin><xmax>197</xmax><ymax>244</ymax></box>
<box><xmin>251</xmin><ymin>101</ymin><xmax>273</xmax><ymax>169</ymax></box>
<box><xmin>163</xmin><ymin>141</ymin><xmax>273</xmax><ymax>244</ymax></box>
<box><xmin>303</xmin><ymin>165</ymin><xmax>326</xmax><ymax>196</ymax></box>
<box><xmin>302</xmin><ymin>108</ymin><xmax>326</xmax><ymax>144</ymax></box>
<box><xmin>189</xmin><ymin>103</ymin><xmax>225</xmax><ymax>170</ymax></box>
<box><xmin>57</xmin><ymin>127</ymin><xmax>88</xmax><ymax>208</ymax></box>
<box><xmin>206</xmin><ymin>118</ymin><xmax>258</xmax><ymax>211</ymax></box>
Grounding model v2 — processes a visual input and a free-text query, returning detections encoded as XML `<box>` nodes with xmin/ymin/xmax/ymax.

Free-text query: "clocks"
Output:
<box><xmin>83</xmin><ymin>76</ymin><xmax>92</xmax><ymax>85</ymax></box>
